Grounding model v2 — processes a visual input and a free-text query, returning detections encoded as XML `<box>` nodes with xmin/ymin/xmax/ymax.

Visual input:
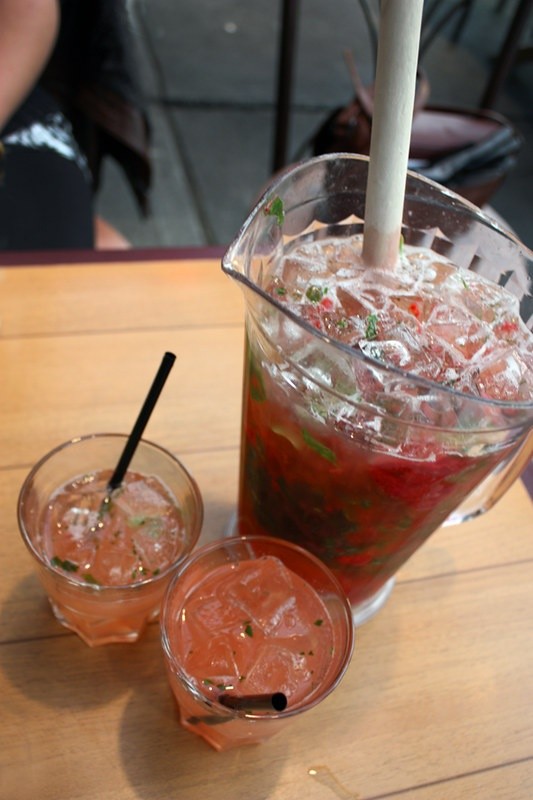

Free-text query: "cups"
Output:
<box><xmin>156</xmin><ymin>534</ymin><xmax>356</xmax><ymax>749</ymax></box>
<box><xmin>16</xmin><ymin>430</ymin><xmax>204</xmax><ymax>646</ymax></box>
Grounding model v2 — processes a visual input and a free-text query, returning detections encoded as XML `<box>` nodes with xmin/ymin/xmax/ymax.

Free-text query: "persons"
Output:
<box><xmin>0</xmin><ymin>0</ymin><xmax>154</xmax><ymax>250</ymax></box>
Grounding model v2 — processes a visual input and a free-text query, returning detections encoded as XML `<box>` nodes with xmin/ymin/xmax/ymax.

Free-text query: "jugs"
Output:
<box><xmin>214</xmin><ymin>147</ymin><xmax>531</xmax><ymax>644</ymax></box>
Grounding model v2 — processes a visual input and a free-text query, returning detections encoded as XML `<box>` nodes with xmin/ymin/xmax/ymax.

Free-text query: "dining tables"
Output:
<box><xmin>1</xmin><ymin>246</ymin><xmax>532</xmax><ymax>799</ymax></box>
